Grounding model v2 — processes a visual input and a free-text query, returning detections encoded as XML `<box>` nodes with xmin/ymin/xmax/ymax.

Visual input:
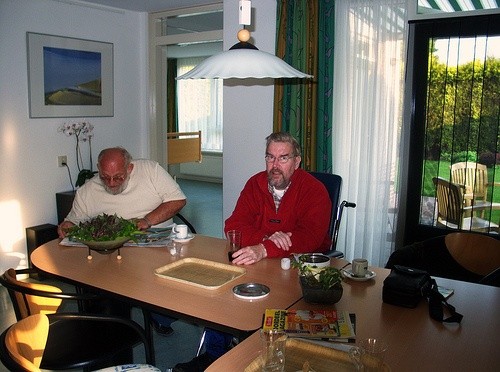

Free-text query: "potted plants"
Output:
<box><xmin>290</xmin><ymin>257</ymin><xmax>346</xmax><ymax>306</ymax></box>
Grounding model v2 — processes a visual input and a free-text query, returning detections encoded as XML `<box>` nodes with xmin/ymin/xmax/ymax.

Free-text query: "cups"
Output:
<box><xmin>172</xmin><ymin>224</ymin><xmax>188</xmax><ymax>239</ymax></box>
<box><xmin>259</xmin><ymin>328</ymin><xmax>289</xmax><ymax>372</ymax></box>
<box><xmin>352</xmin><ymin>258</ymin><xmax>368</xmax><ymax>277</ymax></box>
<box><xmin>227</xmin><ymin>230</ymin><xmax>242</xmax><ymax>263</ymax></box>
<box><xmin>167</xmin><ymin>242</ymin><xmax>184</xmax><ymax>260</ymax></box>
<box><xmin>348</xmin><ymin>336</ymin><xmax>391</xmax><ymax>372</ymax></box>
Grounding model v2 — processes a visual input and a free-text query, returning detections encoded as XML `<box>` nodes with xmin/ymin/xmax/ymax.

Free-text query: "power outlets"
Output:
<box><xmin>58</xmin><ymin>156</ymin><xmax>67</xmax><ymax>166</ymax></box>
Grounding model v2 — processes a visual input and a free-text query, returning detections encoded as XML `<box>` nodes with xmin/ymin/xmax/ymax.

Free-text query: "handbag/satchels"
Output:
<box><xmin>381</xmin><ymin>263</ymin><xmax>434</xmax><ymax>308</ymax></box>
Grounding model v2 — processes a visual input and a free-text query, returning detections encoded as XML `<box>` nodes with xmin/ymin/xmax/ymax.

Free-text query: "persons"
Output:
<box><xmin>173</xmin><ymin>132</ymin><xmax>332</xmax><ymax>372</ymax></box>
<box><xmin>57</xmin><ymin>147</ymin><xmax>187</xmax><ymax>335</ymax></box>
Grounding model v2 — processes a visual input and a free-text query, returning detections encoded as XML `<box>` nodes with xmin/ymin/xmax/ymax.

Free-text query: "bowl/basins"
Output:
<box><xmin>298</xmin><ymin>254</ymin><xmax>331</xmax><ymax>282</ymax></box>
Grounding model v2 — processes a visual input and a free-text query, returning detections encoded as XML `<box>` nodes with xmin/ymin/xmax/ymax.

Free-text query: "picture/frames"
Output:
<box><xmin>26</xmin><ymin>31</ymin><xmax>115</xmax><ymax>119</ymax></box>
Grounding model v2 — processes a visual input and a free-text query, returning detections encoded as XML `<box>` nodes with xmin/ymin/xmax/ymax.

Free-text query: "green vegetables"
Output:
<box><xmin>61</xmin><ymin>213</ymin><xmax>148</xmax><ymax>247</ymax></box>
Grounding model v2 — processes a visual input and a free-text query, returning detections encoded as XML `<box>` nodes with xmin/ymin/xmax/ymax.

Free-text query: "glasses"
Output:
<box><xmin>264</xmin><ymin>155</ymin><xmax>296</xmax><ymax>163</ymax></box>
<box><xmin>99</xmin><ymin>167</ymin><xmax>129</xmax><ymax>182</ymax></box>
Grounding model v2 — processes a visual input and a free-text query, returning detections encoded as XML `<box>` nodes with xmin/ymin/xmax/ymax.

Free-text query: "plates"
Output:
<box><xmin>170</xmin><ymin>234</ymin><xmax>194</xmax><ymax>243</ymax></box>
<box><xmin>344</xmin><ymin>268</ymin><xmax>376</xmax><ymax>281</ymax></box>
<box><xmin>232</xmin><ymin>282</ymin><xmax>270</xmax><ymax>300</ymax></box>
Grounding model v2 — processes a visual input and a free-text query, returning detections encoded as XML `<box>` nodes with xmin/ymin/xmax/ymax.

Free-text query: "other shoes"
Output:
<box><xmin>147</xmin><ymin>322</ymin><xmax>174</xmax><ymax>336</ymax></box>
<box><xmin>174</xmin><ymin>351</ymin><xmax>218</xmax><ymax>372</ymax></box>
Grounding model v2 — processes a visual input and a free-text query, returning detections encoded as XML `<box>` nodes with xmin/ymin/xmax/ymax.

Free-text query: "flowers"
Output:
<box><xmin>57</xmin><ymin>120</ymin><xmax>99</xmax><ymax>187</ymax></box>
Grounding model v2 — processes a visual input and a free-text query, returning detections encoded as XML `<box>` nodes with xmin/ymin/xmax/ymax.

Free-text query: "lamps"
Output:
<box><xmin>175</xmin><ymin>0</ymin><xmax>314</xmax><ymax>80</ymax></box>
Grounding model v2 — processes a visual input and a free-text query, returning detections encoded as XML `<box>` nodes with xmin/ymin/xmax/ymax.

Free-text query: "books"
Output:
<box><xmin>263</xmin><ymin>309</ymin><xmax>356</xmax><ymax>343</ymax></box>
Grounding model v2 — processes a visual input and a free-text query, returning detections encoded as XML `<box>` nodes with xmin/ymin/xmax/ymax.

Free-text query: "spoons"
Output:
<box><xmin>340</xmin><ymin>268</ymin><xmax>358</xmax><ymax>278</ymax></box>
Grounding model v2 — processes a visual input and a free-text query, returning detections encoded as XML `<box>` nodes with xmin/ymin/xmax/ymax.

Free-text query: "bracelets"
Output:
<box><xmin>144</xmin><ymin>217</ymin><xmax>151</xmax><ymax>226</ymax></box>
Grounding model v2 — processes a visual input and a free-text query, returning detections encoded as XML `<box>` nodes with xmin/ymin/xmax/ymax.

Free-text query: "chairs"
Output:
<box><xmin>0</xmin><ymin>268</ymin><xmax>161</xmax><ymax>372</ymax></box>
<box><xmin>386</xmin><ymin>161</ymin><xmax>500</xmax><ymax>288</ymax></box>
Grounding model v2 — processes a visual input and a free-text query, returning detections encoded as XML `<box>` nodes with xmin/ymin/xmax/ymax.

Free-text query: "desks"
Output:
<box><xmin>31</xmin><ymin>237</ymin><xmax>500</xmax><ymax>372</ymax></box>
<box><xmin>56</xmin><ymin>191</ymin><xmax>76</xmax><ymax>224</ymax></box>
